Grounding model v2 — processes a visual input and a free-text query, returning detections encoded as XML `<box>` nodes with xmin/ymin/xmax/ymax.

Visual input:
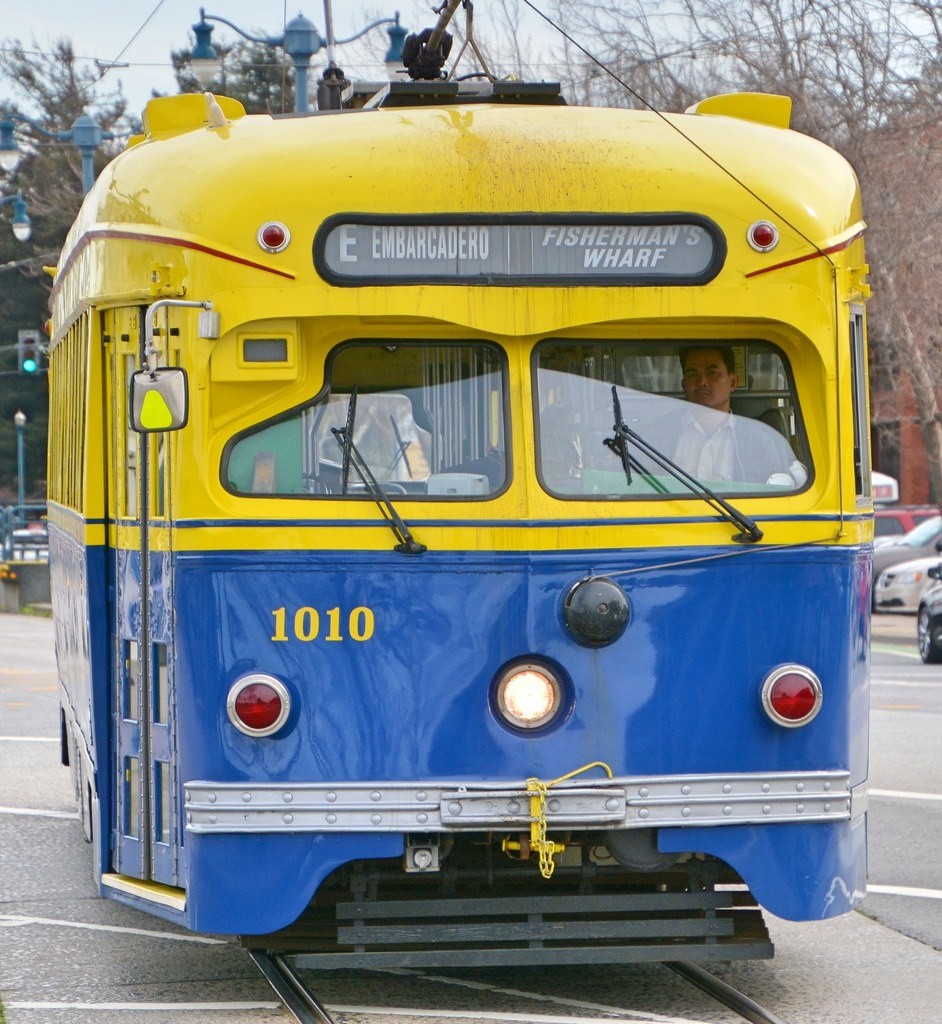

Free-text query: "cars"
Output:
<box><xmin>871</xmin><ymin>504</ymin><xmax>942</xmax><ymax>662</ymax></box>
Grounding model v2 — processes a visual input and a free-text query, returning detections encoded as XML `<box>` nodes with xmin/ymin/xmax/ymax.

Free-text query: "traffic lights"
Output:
<box><xmin>19</xmin><ymin>328</ymin><xmax>41</xmax><ymax>375</ymax></box>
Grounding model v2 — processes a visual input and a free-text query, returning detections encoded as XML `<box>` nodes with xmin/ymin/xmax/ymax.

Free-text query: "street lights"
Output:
<box><xmin>14</xmin><ymin>410</ymin><xmax>26</xmax><ymax>530</ymax></box>
<box><xmin>189</xmin><ymin>7</ymin><xmax>411</xmax><ymax>115</ymax></box>
<box><xmin>1</xmin><ymin>114</ymin><xmax>144</xmax><ymax>200</ymax></box>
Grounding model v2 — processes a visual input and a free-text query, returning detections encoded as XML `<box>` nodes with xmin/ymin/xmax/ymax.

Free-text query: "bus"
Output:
<box><xmin>45</xmin><ymin>0</ymin><xmax>873</xmax><ymax>971</ymax></box>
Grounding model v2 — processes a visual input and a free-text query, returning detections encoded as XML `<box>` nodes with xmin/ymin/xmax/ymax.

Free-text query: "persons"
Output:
<box><xmin>638</xmin><ymin>344</ymin><xmax>784</xmax><ymax>484</ymax></box>
<box><xmin>540</xmin><ymin>382</ymin><xmax>583</xmax><ymax>488</ymax></box>
